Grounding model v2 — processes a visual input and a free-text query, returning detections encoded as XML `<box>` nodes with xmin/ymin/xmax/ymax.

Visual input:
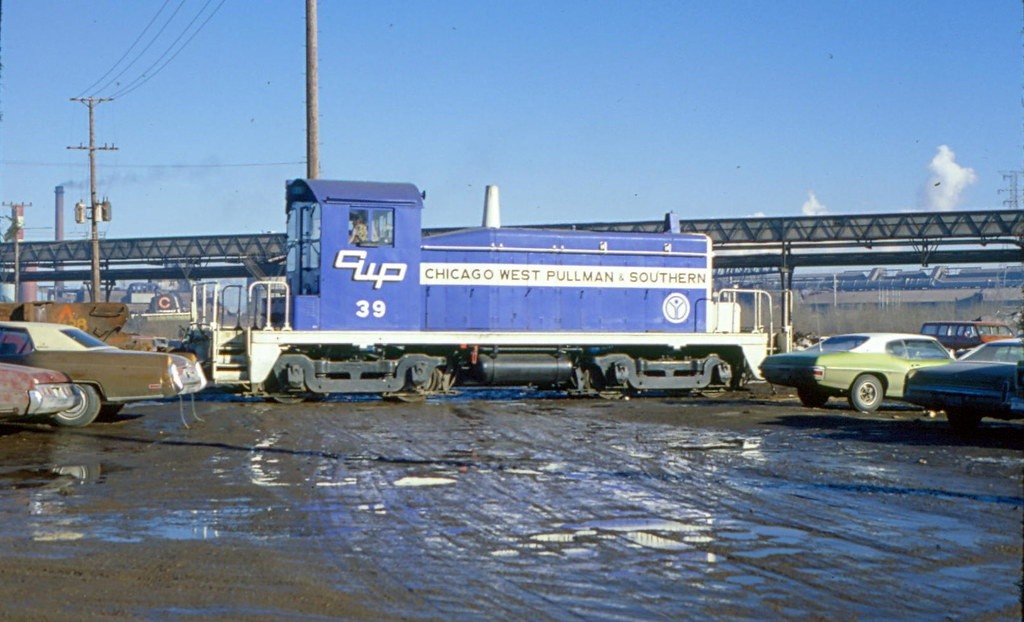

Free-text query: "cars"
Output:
<box><xmin>759</xmin><ymin>332</ymin><xmax>957</xmax><ymax>415</ymax></box>
<box><xmin>1</xmin><ymin>322</ymin><xmax>208</xmax><ymax>427</ymax></box>
<box><xmin>904</xmin><ymin>339</ymin><xmax>1024</xmax><ymax>430</ymax></box>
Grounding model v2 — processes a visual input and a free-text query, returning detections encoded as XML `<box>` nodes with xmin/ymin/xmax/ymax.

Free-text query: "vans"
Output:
<box><xmin>920</xmin><ymin>320</ymin><xmax>1017</xmax><ymax>352</ymax></box>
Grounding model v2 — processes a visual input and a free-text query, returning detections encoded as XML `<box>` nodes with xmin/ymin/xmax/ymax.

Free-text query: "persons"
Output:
<box><xmin>348</xmin><ymin>214</ymin><xmax>367</xmax><ymax>245</ymax></box>
